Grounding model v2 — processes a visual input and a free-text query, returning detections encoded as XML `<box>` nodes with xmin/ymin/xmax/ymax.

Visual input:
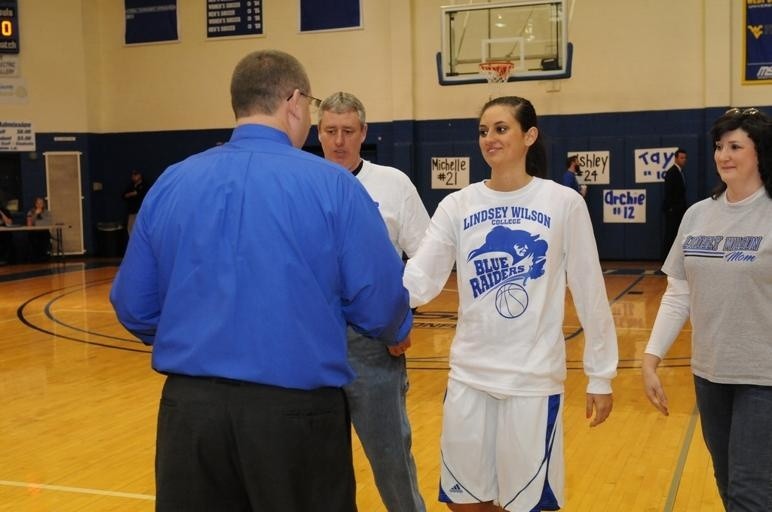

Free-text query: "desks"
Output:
<box><xmin>0</xmin><ymin>225</ymin><xmax>71</xmax><ymax>264</ymax></box>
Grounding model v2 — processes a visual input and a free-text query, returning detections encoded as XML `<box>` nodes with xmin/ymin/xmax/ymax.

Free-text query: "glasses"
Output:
<box><xmin>286</xmin><ymin>88</ymin><xmax>322</xmax><ymax>114</ymax></box>
<box><xmin>724</xmin><ymin>106</ymin><xmax>761</xmax><ymax>121</ymax></box>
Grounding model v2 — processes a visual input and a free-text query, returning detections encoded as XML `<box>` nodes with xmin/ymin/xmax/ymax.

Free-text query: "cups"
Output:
<box><xmin>28</xmin><ymin>216</ymin><xmax>33</xmax><ymax>226</ymax></box>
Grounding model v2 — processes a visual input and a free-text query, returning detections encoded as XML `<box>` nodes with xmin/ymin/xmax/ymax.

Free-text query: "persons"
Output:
<box><xmin>403</xmin><ymin>95</ymin><xmax>620</xmax><ymax>511</ymax></box>
<box><xmin>27</xmin><ymin>196</ymin><xmax>53</xmax><ymax>227</ymax></box>
<box><xmin>318</xmin><ymin>92</ymin><xmax>432</xmax><ymax>511</ymax></box>
<box><xmin>642</xmin><ymin>108</ymin><xmax>771</xmax><ymax>511</ymax></box>
<box><xmin>120</xmin><ymin>167</ymin><xmax>147</xmax><ymax>236</ymax></box>
<box><xmin>0</xmin><ymin>201</ymin><xmax>12</xmax><ymax>226</ymax></box>
<box><xmin>111</xmin><ymin>49</ymin><xmax>414</xmax><ymax>512</ymax></box>
<box><xmin>561</xmin><ymin>156</ymin><xmax>587</xmax><ymax>198</ymax></box>
<box><xmin>659</xmin><ymin>148</ymin><xmax>689</xmax><ymax>266</ymax></box>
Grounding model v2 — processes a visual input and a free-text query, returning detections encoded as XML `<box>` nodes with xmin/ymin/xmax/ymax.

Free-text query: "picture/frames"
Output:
<box><xmin>741</xmin><ymin>0</ymin><xmax>772</xmax><ymax>85</ymax></box>
<box><xmin>123</xmin><ymin>0</ymin><xmax>181</xmax><ymax>46</ymax></box>
<box><xmin>297</xmin><ymin>0</ymin><xmax>364</xmax><ymax>34</ymax></box>
<box><xmin>204</xmin><ymin>0</ymin><xmax>265</xmax><ymax>41</ymax></box>
